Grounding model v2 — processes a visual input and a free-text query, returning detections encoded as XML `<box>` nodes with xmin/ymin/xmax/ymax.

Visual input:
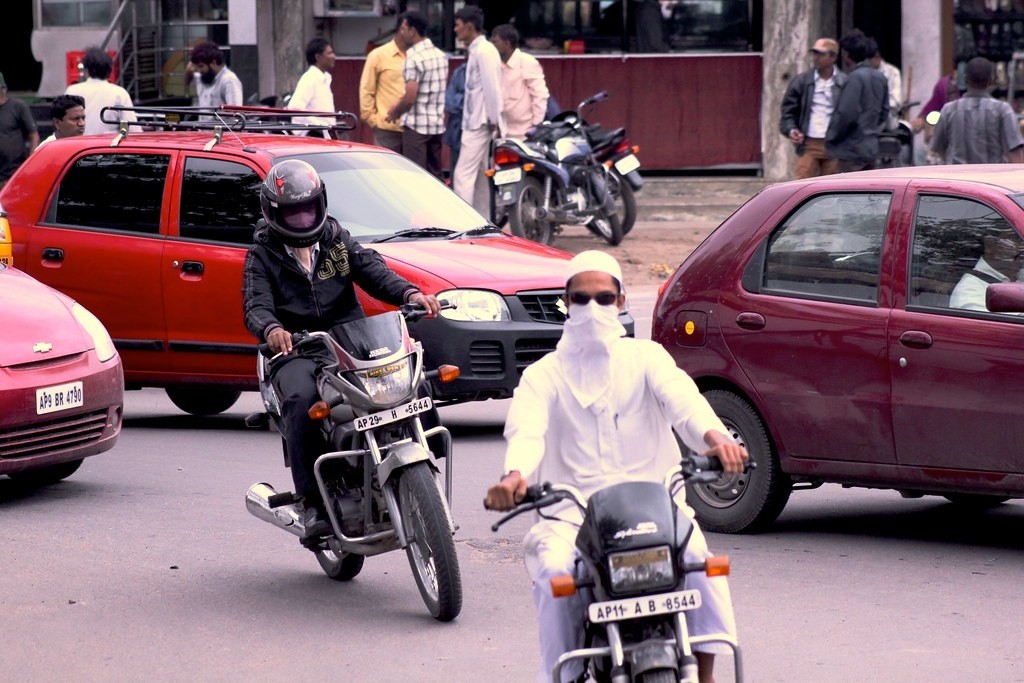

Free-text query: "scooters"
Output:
<box><xmin>878</xmin><ymin>100</ymin><xmax>920</xmax><ymax>166</ymax></box>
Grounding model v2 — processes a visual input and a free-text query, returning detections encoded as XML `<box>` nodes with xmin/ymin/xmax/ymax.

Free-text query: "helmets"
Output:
<box><xmin>260</xmin><ymin>159</ymin><xmax>327</xmax><ymax>248</ymax></box>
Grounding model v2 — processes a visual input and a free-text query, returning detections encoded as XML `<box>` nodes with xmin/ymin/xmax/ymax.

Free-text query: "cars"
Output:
<box><xmin>652</xmin><ymin>163</ymin><xmax>1024</xmax><ymax>534</ymax></box>
<box><xmin>1</xmin><ymin>258</ymin><xmax>126</xmax><ymax>485</ymax></box>
<box><xmin>0</xmin><ymin>103</ymin><xmax>636</xmax><ymax>417</ymax></box>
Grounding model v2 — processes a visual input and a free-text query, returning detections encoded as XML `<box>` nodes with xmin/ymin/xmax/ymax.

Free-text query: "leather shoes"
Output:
<box><xmin>304</xmin><ymin>507</ymin><xmax>329</xmax><ymax>536</ymax></box>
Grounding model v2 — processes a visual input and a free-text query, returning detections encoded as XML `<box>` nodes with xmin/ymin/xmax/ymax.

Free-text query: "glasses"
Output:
<box><xmin>567</xmin><ymin>291</ymin><xmax>619</xmax><ymax>305</ymax></box>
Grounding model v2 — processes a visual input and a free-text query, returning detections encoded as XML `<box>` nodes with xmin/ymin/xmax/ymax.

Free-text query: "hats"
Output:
<box><xmin>566</xmin><ymin>251</ymin><xmax>622</xmax><ymax>284</ymax></box>
<box><xmin>808</xmin><ymin>38</ymin><xmax>839</xmax><ymax>54</ymax></box>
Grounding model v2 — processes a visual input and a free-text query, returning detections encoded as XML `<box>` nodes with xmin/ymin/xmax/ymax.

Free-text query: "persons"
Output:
<box><xmin>360</xmin><ymin>13</ymin><xmax>412</xmax><ymax>155</ymax></box>
<box><xmin>930</xmin><ymin>57</ymin><xmax>1024</xmax><ymax>165</ymax></box>
<box><xmin>948</xmin><ymin>218</ymin><xmax>1024</xmax><ymax>312</ymax></box>
<box><xmin>454</xmin><ymin>6</ymin><xmax>504</xmax><ymax>220</ymax></box>
<box><xmin>486</xmin><ymin>249</ymin><xmax>749</xmax><ymax>683</ymax></box>
<box><xmin>445</xmin><ymin>63</ymin><xmax>497</xmax><ymax>225</ymax></box>
<box><xmin>385</xmin><ymin>10</ymin><xmax>448</xmax><ymax>181</ymax></box>
<box><xmin>636</xmin><ymin>0</ymin><xmax>671</xmax><ymax>53</ymax></box>
<box><xmin>911</xmin><ymin>57</ymin><xmax>967</xmax><ymax>165</ymax></box>
<box><xmin>287</xmin><ymin>38</ymin><xmax>340</xmax><ymax>139</ymax></box>
<box><xmin>242</xmin><ymin>160</ymin><xmax>441</xmax><ymax>545</ymax></box>
<box><xmin>824</xmin><ymin>28</ymin><xmax>902</xmax><ymax>180</ymax></box>
<box><xmin>191</xmin><ymin>41</ymin><xmax>244</xmax><ymax>132</ymax></box>
<box><xmin>778</xmin><ymin>38</ymin><xmax>846</xmax><ymax>179</ymax></box>
<box><xmin>491</xmin><ymin>23</ymin><xmax>550</xmax><ymax>143</ymax></box>
<box><xmin>0</xmin><ymin>73</ymin><xmax>39</xmax><ymax>192</ymax></box>
<box><xmin>34</xmin><ymin>94</ymin><xmax>86</xmax><ymax>152</ymax></box>
<box><xmin>63</xmin><ymin>45</ymin><xmax>143</xmax><ymax>135</ymax></box>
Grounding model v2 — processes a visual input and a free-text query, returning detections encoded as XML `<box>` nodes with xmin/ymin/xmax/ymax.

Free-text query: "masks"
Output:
<box><xmin>284</xmin><ymin>212</ymin><xmax>317</xmax><ymax>229</ymax></box>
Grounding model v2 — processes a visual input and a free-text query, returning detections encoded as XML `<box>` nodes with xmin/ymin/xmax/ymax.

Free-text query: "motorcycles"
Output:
<box><xmin>245</xmin><ymin>299</ymin><xmax>463</xmax><ymax>621</ymax></box>
<box><xmin>484</xmin><ymin>90</ymin><xmax>646</xmax><ymax>245</ymax></box>
<box><xmin>484</xmin><ymin>454</ymin><xmax>743</xmax><ymax>683</ymax></box>
